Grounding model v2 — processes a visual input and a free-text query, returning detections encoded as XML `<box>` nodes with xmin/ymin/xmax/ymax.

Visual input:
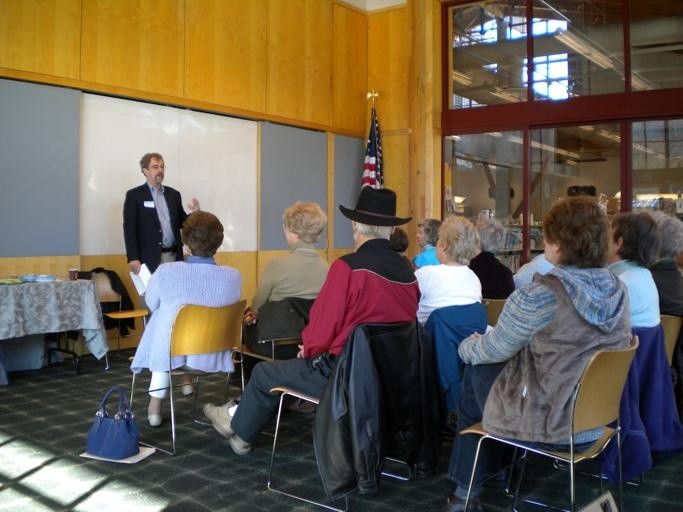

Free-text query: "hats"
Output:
<box><xmin>338</xmin><ymin>185</ymin><xmax>411</xmax><ymax>227</ymax></box>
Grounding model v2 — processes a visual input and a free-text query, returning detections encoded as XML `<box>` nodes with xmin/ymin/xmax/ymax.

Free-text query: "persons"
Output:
<box><xmin>600</xmin><ymin>212</ymin><xmax>659</xmax><ymax>331</ymax></box>
<box><xmin>202</xmin><ymin>187</ymin><xmax>421</xmax><ymax>454</ymax></box>
<box><xmin>230</xmin><ymin>201</ymin><xmax>331</xmax><ymax>389</ymax></box>
<box><xmin>128</xmin><ymin>210</ymin><xmax>241</xmax><ymax>426</ymax></box>
<box><xmin>411</xmin><ymin>219</ymin><xmax>440</xmax><ymax>269</ymax></box>
<box><xmin>446</xmin><ymin>195</ymin><xmax>632</xmax><ymax>511</ymax></box>
<box><xmin>404</xmin><ymin>216</ymin><xmax>481</xmax><ymax>478</ymax></box>
<box><xmin>122</xmin><ymin>153</ymin><xmax>200</xmax><ymax>331</ymax></box>
<box><xmin>643</xmin><ymin>212</ymin><xmax>682</xmax><ymax>317</ymax></box>
<box><xmin>388</xmin><ymin>227</ymin><xmax>417</xmax><ymax>275</ymax></box>
<box><xmin>468</xmin><ymin>219</ymin><xmax>514</xmax><ymax>300</ymax></box>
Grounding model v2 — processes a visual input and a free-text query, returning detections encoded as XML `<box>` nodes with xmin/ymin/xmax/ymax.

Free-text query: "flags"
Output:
<box><xmin>359</xmin><ymin>106</ymin><xmax>383</xmax><ymax>189</ymax></box>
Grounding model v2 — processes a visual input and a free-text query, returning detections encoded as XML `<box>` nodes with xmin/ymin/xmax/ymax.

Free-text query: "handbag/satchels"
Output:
<box><xmin>85</xmin><ymin>386</ymin><xmax>140</xmax><ymax>460</ymax></box>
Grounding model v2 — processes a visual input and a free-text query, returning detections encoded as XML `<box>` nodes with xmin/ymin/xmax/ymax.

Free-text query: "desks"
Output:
<box><xmin>0</xmin><ymin>280</ymin><xmax>95</xmax><ymax>376</ymax></box>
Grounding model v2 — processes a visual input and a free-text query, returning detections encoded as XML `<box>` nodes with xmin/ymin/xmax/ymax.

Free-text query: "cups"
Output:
<box><xmin>69</xmin><ymin>270</ymin><xmax>80</xmax><ymax>281</ymax></box>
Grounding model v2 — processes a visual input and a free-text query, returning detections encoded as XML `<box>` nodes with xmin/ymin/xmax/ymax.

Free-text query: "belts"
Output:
<box><xmin>160</xmin><ymin>247</ymin><xmax>173</xmax><ymax>252</ymax></box>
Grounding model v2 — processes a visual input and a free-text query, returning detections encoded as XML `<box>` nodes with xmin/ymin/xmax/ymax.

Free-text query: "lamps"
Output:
<box><xmin>509</xmin><ymin>136</ymin><xmax>579</xmax><ymax>158</ymax></box>
<box><xmin>487</xmin><ymin>131</ymin><xmax>501</xmax><ymax>136</ymax></box>
<box><xmin>622</xmin><ymin>74</ymin><xmax>654</xmax><ymax>90</ymax></box>
<box><xmin>454</xmin><ymin>196</ymin><xmax>466</xmax><ymax>212</ymax></box>
<box><xmin>453</xmin><ymin>69</ymin><xmax>473</xmax><ymax>86</ymax></box>
<box><xmin>487</xmin><ymin>85</ymin><xmax>522</xmax><ymax>103</ymax></box>
<box><xmin>636</xmin><ymin>194</ymin><xmax>678</xmax><ymax>200</ymax></box>
<box><xmin>553</xmin><ymin>28</ymin><xmax>614</xmax><ymax>70</ymax></box>
<box><xmin>579</xmin><ymin>125</ymin><xmax>655</xmax><ymax>154</ymax></box>
<box><xmin>444</xmin><ymin>135</ymin><xmax>461</xmax><ymax>141</ymax></box>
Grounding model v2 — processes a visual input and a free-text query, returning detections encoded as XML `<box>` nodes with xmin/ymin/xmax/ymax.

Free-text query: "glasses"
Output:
<box><xmin>148</xmin><ymin>165</ymin><xmax>166</xmax><ymax>170</ymax></box>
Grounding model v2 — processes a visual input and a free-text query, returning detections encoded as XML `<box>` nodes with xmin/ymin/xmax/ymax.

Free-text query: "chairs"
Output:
<box><xmin>459</xmin><ymin>336</ymin><xmax>639</xmax><ymax>512</ymax></box>
<box><xmin>266</xmin><ymin>322</ymin><xmax>416</xmax><ymax>512</ymax></box>
<box><xmin>90</xmin><ymin>270</ymin><xmax>149</xmax><ymax>369</ymax></box>
<box><xmin>127</xmin><ymin>300</ymin><xmax>248</xmax><ymax>456</ymax></box>
<box><xmin>225</xmin><ymin>298</ymin><xmax>314</xmax><ymax>435</ymax></box>
<box><xmin>482</xmin><ymin>298</ymin><xmax>507</xmax><ymax>326</ymax></box>
<box><xmin>659</xmin><ymin>315</ymin><xmax>683</xmax><ymax>367</ymax></box>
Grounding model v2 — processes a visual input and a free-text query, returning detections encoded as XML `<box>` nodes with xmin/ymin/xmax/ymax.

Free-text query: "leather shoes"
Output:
<box><xmin>202</xmin><ymin>402</ymin><xmax>234</xmax><ymax>439</ymax></box>
<box><xmin>181</xmin><ymin>384</ymin><xmax>192</xmax><ymax>395</ymax></box>
<box><xmin>228</xmin><ymin>434</ymin><xmax>252</xmax><ymax>455</ymax></box>
<box><xmin>436</xmin><ymin>497</ymin><xmax>481</xmax><ymax>512</ymax></box>
<box><xmin>147</xmin><ymin>414</ymin><xmax>162</xmax><ymax>427</ymax></box>
